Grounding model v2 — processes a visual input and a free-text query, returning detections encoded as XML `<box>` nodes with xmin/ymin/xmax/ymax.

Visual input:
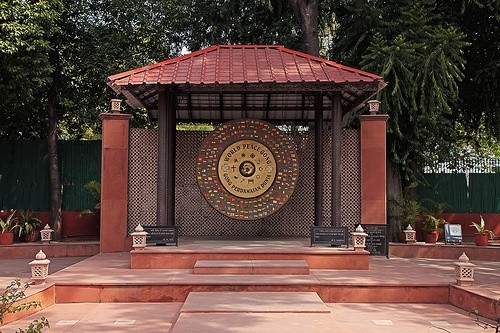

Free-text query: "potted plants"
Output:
<box><xmin>420</xmin><ymin>198</ymin><xmax>447</xmax><ymax>243</ymax></box>
<box><xmin>11</xmin><ymin>207</ymin><xmax>43</xmax><ymax>241</ymax></box>
<box><xmin>0</xmin><ymin>207</ymin><xmax>17</xmax><ymax>245</ymax></box>
<box><xmin>469</xmin><ymin>213</ymin><xmax>495</xmax><ymax>245</ymax></box>
